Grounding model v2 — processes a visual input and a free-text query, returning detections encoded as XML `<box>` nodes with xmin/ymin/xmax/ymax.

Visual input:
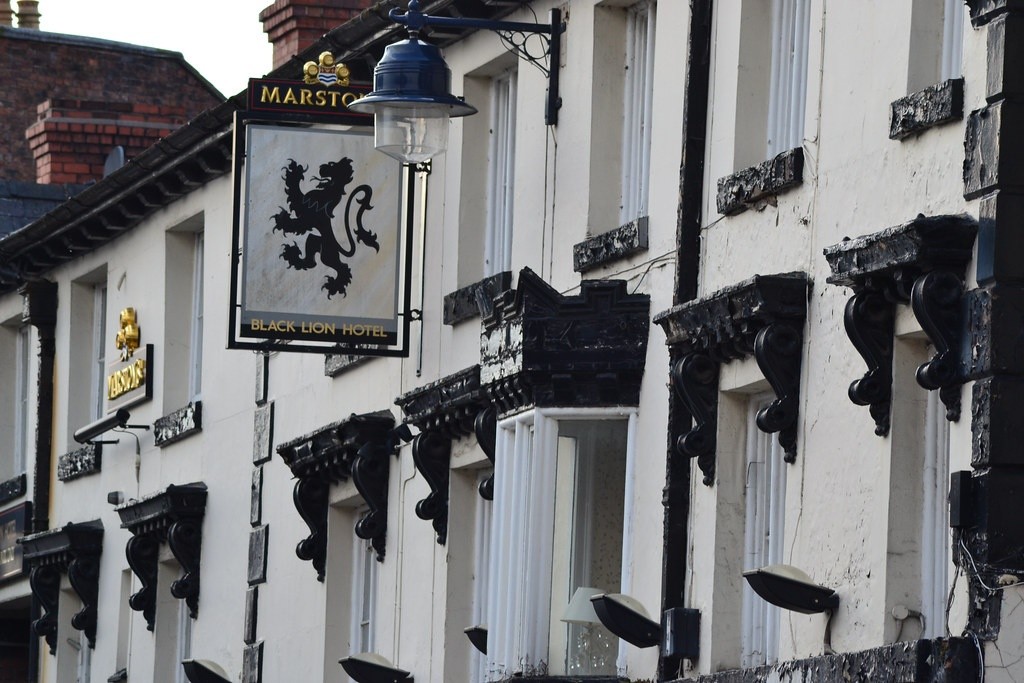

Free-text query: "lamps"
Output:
<box><xmin>559</xmin><ymin>588</ymin><xmax>610</xmax><ymax>675</ymax></box>
<box><xmin>73</xmin><ymin>409</ymin><xmax>150</xmax><ymax>446</ymax></box>
<box><xmin>463</xmin><ymin>625</ymin><xmax>488</xmax><ymax>655</ymax></box>
<box><xmin>590</xmin><ymin>593</ymin><xmax>663</xmax><ymax>648</ymax></box>
<box><xmin>180</xmin><ymin>658</ymin><xmax>233</xmax><ymax>683</ymax></box>
<box><xmin>741</xmin><ymin>563</ymin><xmax>839</xmax><ymax>618</ymax></box>
<box><xmin>336</xmin><ymin>651</ymin><xmax>414</xmax><ymax>683</ymax></box>
<box><xmin>350</xmin><ymin>0</ymin><xmax>566</xmax><ymax>166</ymax></box>
